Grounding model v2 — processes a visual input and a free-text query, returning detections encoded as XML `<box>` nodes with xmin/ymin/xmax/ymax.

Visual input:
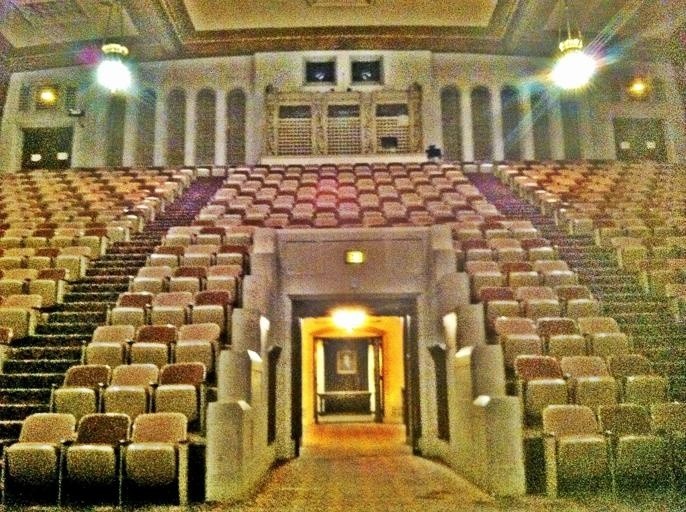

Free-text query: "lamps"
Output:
<box><xmin>95</xmin><ymin>0</ymin><xmax>132</xmax><ymax>91</ymax></box>
<box><xmin>551</xmin><ymin>1</ymin><xmax>596</xmax><ymax>90</ymax></box>
